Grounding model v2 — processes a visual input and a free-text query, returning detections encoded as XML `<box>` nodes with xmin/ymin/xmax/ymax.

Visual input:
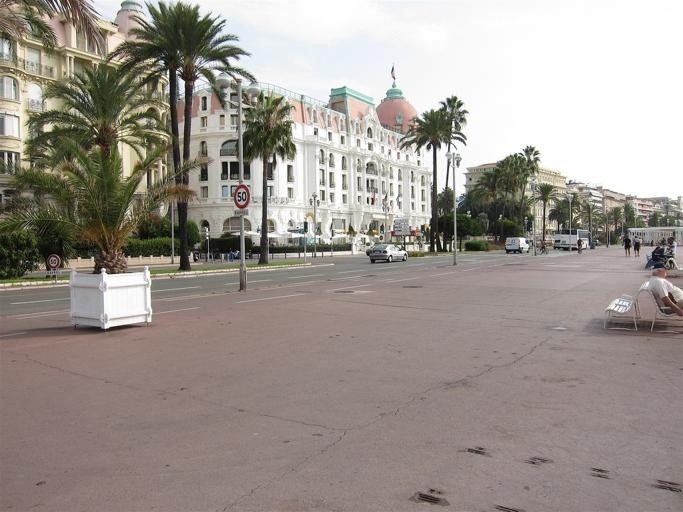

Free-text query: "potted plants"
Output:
<box><xmin>0</xmin><ymin>63</ymin><xmax>212</xmax><ymax>331</ymax></box>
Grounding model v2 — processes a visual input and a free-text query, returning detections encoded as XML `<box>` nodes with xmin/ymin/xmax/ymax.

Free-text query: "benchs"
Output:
<box><xmin>602</xmin><ymin>280</ymin><xmax>682</xmax><ymax>334</ymax></box>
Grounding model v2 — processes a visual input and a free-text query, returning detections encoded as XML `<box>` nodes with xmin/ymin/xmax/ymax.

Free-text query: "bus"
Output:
<box><xmin>554</xmin><ymin>228</ymin><xmax>588</xmax><ymax>249</ymax></box>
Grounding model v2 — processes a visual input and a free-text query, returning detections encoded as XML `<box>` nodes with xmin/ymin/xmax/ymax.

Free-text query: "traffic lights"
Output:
<box><xmin>380</xmin><ymin>224</ymin><xmax>384</xmax><ymax>233</ymax></box>
<box><xmin>304</xmin><ymin>221</ymin><xmax>307</xmax><ymax>232</ymax></box>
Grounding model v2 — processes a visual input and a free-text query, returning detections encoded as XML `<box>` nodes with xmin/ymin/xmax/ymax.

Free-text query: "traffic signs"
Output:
<box><xmin>234</xmin><ymin>184</ymin><xmax>250</xmax><ymax>209</ymax></box>
<box><xmin>47</xmin><ymin>254</ymin><xmax>61</xmax><ymax>269</ymax></box>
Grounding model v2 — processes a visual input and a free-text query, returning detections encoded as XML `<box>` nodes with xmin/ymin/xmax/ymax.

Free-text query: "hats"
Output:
<box><xmin>654</xmin><ymin>263</ymin><xmax>669</xmax><ymax>270</ymax></box>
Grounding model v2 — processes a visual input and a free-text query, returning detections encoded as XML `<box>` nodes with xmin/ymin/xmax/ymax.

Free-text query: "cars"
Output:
<box><xmin>366</xmin><ymin>242</ymin><xmax>407</xmax><ymax>263</ymax></box>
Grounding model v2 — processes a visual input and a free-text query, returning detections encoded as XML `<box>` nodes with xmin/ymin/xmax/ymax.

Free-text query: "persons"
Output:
<box><xmin>665</xmin><ymin>236</ymin><xmax>677</xmax><ymax>259</ymax></box>
<box><xmin>622</xmin><ymin>233</ymin><xmax>631</xmax><ymax>256</ymax></box>
<box><xmin>661</xmin><ymin>237</ymin><xmax>666</xmax><ymax>245</ymax></box>
<box><xmin>539</xmin><ymin>238</ymin><xmax>548</xmax><ymax>254</ymax></box>
<box><xmin>639</xmin><ymin>237</ymin><xmax>642</xmax><ymax>244</ymax></box>
<box><xmin>633</xmin><ymin>235</ymin><xmax>640</xmax><ymax>257</ymax></box>
<box><xmin>649</xmin><ymin>262</ymin><xmax>683</xmax><ymax>316</ymax></box>
<box><xmin>650</xmin><ymin>246</ymin><xmax>683</xmax><ymax>271</ymax></box>
<box><xmin>577</xmin><ymin>238</ymin><xmax>585</xmax><ymax>247</ymax></box>
<box><xmin>651</xmin><ymin>239</ymin><xmax>654</xmax><ymax>246</ymax></box>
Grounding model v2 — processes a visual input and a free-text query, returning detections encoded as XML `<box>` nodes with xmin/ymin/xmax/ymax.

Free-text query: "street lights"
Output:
<box><xmin>530</xmin><ymin>179</ymin><xmax>539</xmax><ymax>255</ymax></box>
<box><xmin>383</xmin><ymin>197</ymin><xmax>392</xmax><ymax>243</ymax></box>
<box><xmin>310</xmin><ymin>192</ymin><xmax>320</xmax><ymax>258</ymax></box>
<box><xmin>447</xmin><ymin>150</ymin><xmax>462</xmax><ymax>265</ymax></box>
<box><xmin>215</xmin><ymin>75</ymin><xmax>260</xmax><ymax>292</ymax></box>
<box><xmin>567</xmin><ymin>193</ymin><xmax>575</xmax><ymax>250</ymax></box>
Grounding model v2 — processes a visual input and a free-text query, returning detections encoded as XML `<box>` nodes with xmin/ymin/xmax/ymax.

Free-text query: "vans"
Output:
<box><xmin>505</xmin><ymin>236</ymin><xmax>530</xmax><ymax>253</ymax></box>
